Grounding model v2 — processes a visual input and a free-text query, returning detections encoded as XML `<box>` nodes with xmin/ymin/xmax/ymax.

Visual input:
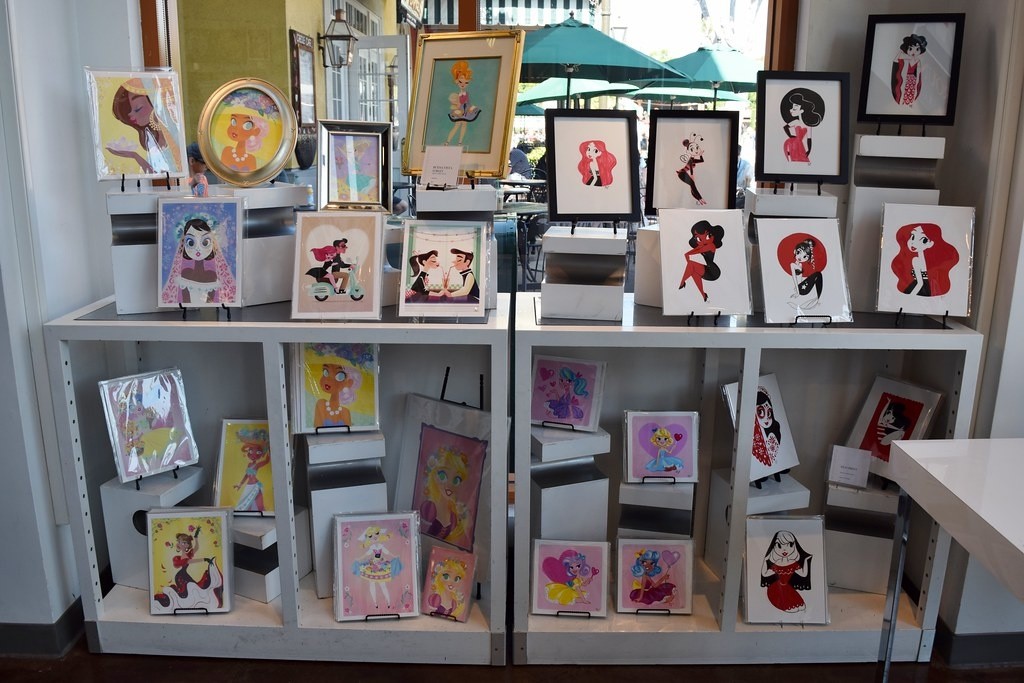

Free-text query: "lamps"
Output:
<box><xmin>316</xmin><ymin>7</ymin><xmax>357</xmax><ymax>69</ymax></box>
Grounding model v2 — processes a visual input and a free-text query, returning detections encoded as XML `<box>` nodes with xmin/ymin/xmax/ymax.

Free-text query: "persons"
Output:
<box><xmin>640</xmin><ymin>134</ymin><xmax>649</xmax><ymax>151</ymax></box>
<box><xmin>393</xmin><ymin>196</ymin><xmax>408</xmax><ymax>217</ymax></box>
<box><xmin>736</xmin><ymin>144</ymin><xmax>754</xmax><ymax>193</ymax></box>
<box><xmin>504</xmin><ymin>149</ymin><xmax>551</xmax><ymax>202</ymax></box>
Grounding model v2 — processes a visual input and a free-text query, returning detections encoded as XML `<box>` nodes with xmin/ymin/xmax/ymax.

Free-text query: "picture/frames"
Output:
<box><xmin>289</xmin><ymin>28</ymin><xmax>318</xmax><ymax>136</ymax></box>
<box><xmin>97</xmin><ymin>366</ymin><xmax>198</xmax><ymax>485</ymax></box>
<box><xmin>544</xmin><ymin>108</ymin><xmax>641</xmax><ymax>224</ymax></box>
<box><xmin>645</xmin><ymin>109</ymin><xmax>740</xmax><ymax>217</ymax></box>
<box><xmin>291</xmin><ymin>211</ymin><xmax>382</xmax><ymax>320</ymax></box>
<box><xmin>214</xmin><ymin>419</ymin><xmax>279</xmax><ymax>516</ymax></box>
<box><xmin>401</xmin><ymin>29</ymin><xmax>526</xmax><ymax>179</ymax></box>
<box><xmin>617</xmin><ymin>538</ymin><xmax>694</xmax><ymax>616</ymax></box>
<box><xmin>331</xmin><ymin>509</ymin><xmax>421</xmax><ymax>622</ymax></box>
<box><xmin>529</xmin><ymin>353</ymin><xmax>608</xmax><ymax>432</ymax></box>
<box><xmin>390</xmin><ymin>392</ymin><xmax>511</xmax><ymax>585</ymax></box>
<box><xmin>399</xmin><ymin>219</ymin><xmax>487</xmax><ymax>318</ymax></box>
<box><xmin>532</xmin><ymin>538</ymin><xmax>608</xmax><ymax>617</ymax></box>
<box><xmin>144</xmin><ymin>505</ymin><xmax>233</xmax><ymax>615</ymax></box>
<box><xmin>195</xmin><ymin>77</ymin><xmax>299</xmax><ymax>188</ymax></box>
<box><xmin>755</xmin><ymin>70</ymin><xmax>852</xmax><ymax>185</ymax></box>
<box><xmin>157</xmin><ymin>195</ymin><xmax>241</xmax><ymax>308</ymax></box>
<box><xmin>855</xmin><ymin>12</ymin><xmax>967</xmax><ymax>127</ymax></box>
<box><xmin>316</xmin><ymin>118</ymin><xmax>393</xmax><ymax>216</ymax></box>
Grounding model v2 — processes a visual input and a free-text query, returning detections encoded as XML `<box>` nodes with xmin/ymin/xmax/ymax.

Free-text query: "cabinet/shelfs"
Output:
<box><xmin>42</xmin><ymin>292</ymin><xmax>513</xmax><ymax>670</ymax></box>
<box><xmin>515</xmin><ymin>290</ymin><xmax>983</xmax><ymax>667</ymax></box>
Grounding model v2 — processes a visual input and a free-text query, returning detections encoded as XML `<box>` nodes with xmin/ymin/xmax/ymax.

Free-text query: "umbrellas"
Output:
<box><xmin>512</xmin><ymin>10</ymin><xmax>764</xmax><ymax>117</ymax></box>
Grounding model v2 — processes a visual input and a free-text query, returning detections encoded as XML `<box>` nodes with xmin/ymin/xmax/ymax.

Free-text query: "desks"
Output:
<box><xmin>874</xmin><ymin>437</ymin><xmax>1024</xmax><ymax>683</ymax></box>
<box><xmin>499</xmin><ymin>178</ymin><xmax>546</xmax><ymax>202</ymax></box>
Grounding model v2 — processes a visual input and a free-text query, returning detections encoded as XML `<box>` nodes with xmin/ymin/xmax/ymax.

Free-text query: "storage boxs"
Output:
<box><xmin>411</xmin><ymin>182</ymin><xmax>502</xmax><ymax>311</ymax></box>
<box><xmin>819</xmin><ymin>472</ymin><xmax>900</xmax><ymax>595</ymax></box>
<box><xmin>539</xmin><ymin>227</ymin><xmax>626</xmax><ymax>323</ymax></box>
<box><xmin>99</xmin><ymin>465</ymin><xmax>207</xmax><ymax>593</ymax></box>
<box><xmin>303</xmin><ymin>430</ymin><xmax>387</xmax><ymax>600</ymax></box>
<box><xmin>633</xmin><ymin>134</ymin><xmax>947</xmax><ymax>322</ymax></box>
<box><xmin>705</xmin><ymin>466</ymin><xmax>811</xmax><ymax>599</ymax></box>
<box><xmin>617</xmin><ymin>483</ymin><xmax>697</xmax><ymax>540</ymax></box>
<box><xmin>231</xmin><ymin>504</ymin><xmax>315</xmax><ymax>605</ymax></box>
<box><xmin>106</xmin><ymin>180</ymin><xmax>309</xmax><ymax>314</ymax></box>
<box><xmin>528</xmin><ymin>422</ymin><xmax>610</xmax><ymax>542</ymax></box>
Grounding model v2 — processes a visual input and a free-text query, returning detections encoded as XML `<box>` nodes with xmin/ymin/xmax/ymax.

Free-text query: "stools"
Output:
<box><xmin>503</xmin><ymin>187</ymin><xmax>530</xmax><ymax>202</ymax></box>
